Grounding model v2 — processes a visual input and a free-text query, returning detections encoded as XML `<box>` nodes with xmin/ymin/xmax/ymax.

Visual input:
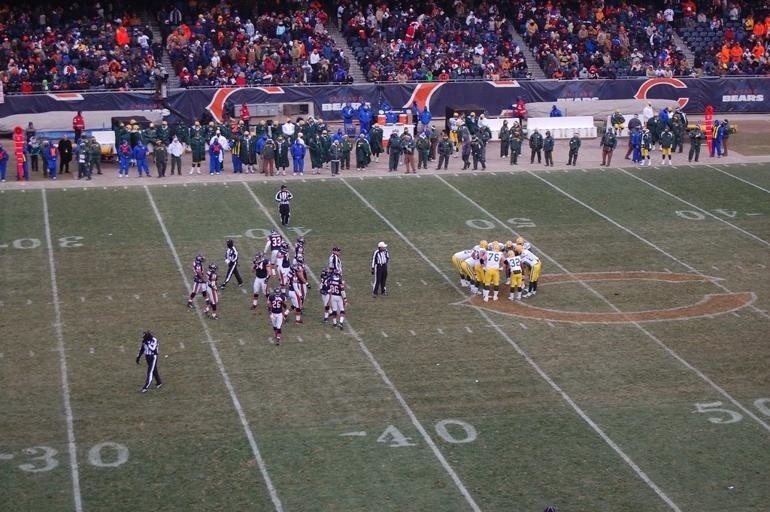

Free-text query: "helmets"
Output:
<box><xmin>480</xmin><ymin>237</ymin><xmax>525</xmax><ymax>258</ymax></box>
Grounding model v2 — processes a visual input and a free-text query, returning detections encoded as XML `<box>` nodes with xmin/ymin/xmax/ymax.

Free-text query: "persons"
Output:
<box><xmin>370</xmin><ymin>241</ymin><xmax>390</xmax><ymax>298</ymax></box>
<box><xmin>274</xmin><ymin>185</ymin><xmax>293</xmax><ymax>226</ymax></box>
<box><xmin>186</xmin><ymin>239</ymin><xmax>242</xmax><ymax>320</ymax></box>
<box><xmin>135</xmin><ymin>328</ymin><xmax>165</xmax><ymax>394</ymax></box>
<box><xmin>452</xmin><ymin>237</ymin><xmax>541</xmax><ymax>302</ymax></box>
<box><xmin>251</xmin><ymin>229</ymin><xmax>348</xmax><ymax>346</ymax></box>
<box><xmin>1</xmin><ymin>0</ymin><xmax>770</xmax><ymax>95</ymax></box>
<box><xmin>1</xmin><ymin>96</ymin><xmax>731</xmax><ymax>181</ymax></box>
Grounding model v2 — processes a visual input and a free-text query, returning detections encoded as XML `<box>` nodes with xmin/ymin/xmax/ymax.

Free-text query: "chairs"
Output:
<box><xmin>0</xmin><ymin>0</ymin><xmax>747</xmax><ymax>87</ymax></box>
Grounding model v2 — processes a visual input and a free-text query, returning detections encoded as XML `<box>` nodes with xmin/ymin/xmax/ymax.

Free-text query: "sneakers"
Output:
<box><xmin>508</xmin><ymin>291</ymin><xmax>538</xmax><ymax>302</ymax></box>
<box><xmin>460</xmin><ymin>282</ymin><xmax>482</xmax><ymax>295</ymax></box>
<box><xmin>323</xmin><ymin>311</ymin><xmax>346</xmax><ymax>330</ymax></box>
<box><xmin>483</xmin><ymin>295</ymin><xmax>499</xmax><ymax>303</ymax></box>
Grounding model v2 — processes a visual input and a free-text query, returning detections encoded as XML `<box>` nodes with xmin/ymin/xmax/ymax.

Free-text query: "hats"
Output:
<box><xmin>378</xmin><ymin>242</ymin><xmax>387</xmax><ymax>247</ymax></box>
